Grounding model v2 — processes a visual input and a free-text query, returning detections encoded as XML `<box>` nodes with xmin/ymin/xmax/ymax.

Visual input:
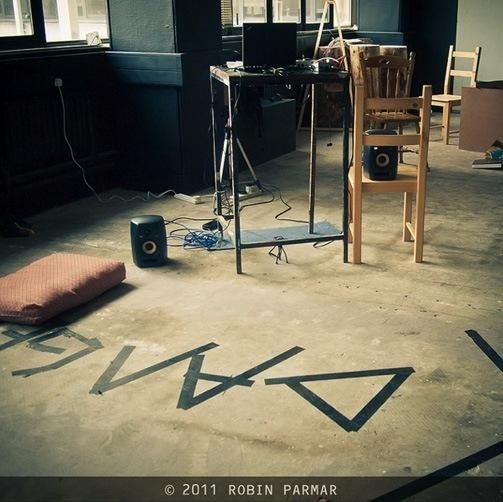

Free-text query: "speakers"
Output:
<box><xmin>365</xmin><ymin>130</ymin><xmax>398</xmax><ymax>180</ymax></box>
<box><xmin>131</xmin><ymin>215</ymin><xmax>167</xmax><ymax>268</ymax></box>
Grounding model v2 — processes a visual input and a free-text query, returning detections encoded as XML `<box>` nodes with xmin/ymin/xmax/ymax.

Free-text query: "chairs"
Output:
<box><xmin>418</xmin><ymin>44</ymin><xmax>481</xmax><ymax>146</ymax></box>
<box><xmin>347</xmin><ymin>84</ymin><xmax>433</xmax><ymax>265</ymax></box>
<box><xmin>358</xmin><ymin>49</ymin><xmax>432</xmax><ymax>172</ymax></box>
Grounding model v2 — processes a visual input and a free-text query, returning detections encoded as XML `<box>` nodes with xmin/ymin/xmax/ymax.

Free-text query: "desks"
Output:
<box><xmin>208</xmin><ymin>66</ymin><xmax>353</xmax><ymax>275</ymax></box>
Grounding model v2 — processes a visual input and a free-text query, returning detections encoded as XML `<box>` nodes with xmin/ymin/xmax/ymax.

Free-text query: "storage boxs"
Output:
<box><xmin>458</xmin><ymin>81</ymin><xmax>503</xmax><ymax>153</ymax></box>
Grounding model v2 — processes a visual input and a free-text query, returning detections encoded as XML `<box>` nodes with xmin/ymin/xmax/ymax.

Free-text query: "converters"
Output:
<box><xmin>246</xmin><ymin>186</ymin><xmax>258</xmax><ymax>193</ymax></box>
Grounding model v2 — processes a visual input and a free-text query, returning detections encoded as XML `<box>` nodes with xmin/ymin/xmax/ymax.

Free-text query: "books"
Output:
<box><xmin>470</xmin><ymin>158</ymin><xmax>501</xmax><ymax>170</ymax></box>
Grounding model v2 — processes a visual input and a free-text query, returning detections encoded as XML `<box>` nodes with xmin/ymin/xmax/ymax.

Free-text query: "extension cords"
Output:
<box><xmin>174</xmin><ymin>193</ymin><xmax>201</xmax><ymax>204</ymax></box>
<box><xmin>238</xmin><ymin>189</ymin><xmax>264</xmax><ymax>200</ymax></box>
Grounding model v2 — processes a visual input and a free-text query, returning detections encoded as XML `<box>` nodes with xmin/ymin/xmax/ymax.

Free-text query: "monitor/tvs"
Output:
<box><xmin>243</xmin><ymin>23</ymin><xmax>297</xmax><ymax>66</ymax></box>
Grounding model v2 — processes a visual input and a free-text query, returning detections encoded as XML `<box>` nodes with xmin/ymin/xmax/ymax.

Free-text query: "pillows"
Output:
<box><xmin>0</xmin><ymin>252</ymin><xmax>125</xmax><ymax>326</ymax></box>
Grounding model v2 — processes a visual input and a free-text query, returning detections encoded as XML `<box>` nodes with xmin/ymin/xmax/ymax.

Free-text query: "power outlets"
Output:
<box><xmin>55</xmin><ymin>78</ymin><xmax>63</xmax><ymax>87</ymax></box>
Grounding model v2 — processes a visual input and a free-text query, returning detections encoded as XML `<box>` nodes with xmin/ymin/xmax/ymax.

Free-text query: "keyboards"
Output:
<box><xmin>245</xmin><ymin>61</ymin><xmax>315</xmax><ymax>73</ymax></box>
